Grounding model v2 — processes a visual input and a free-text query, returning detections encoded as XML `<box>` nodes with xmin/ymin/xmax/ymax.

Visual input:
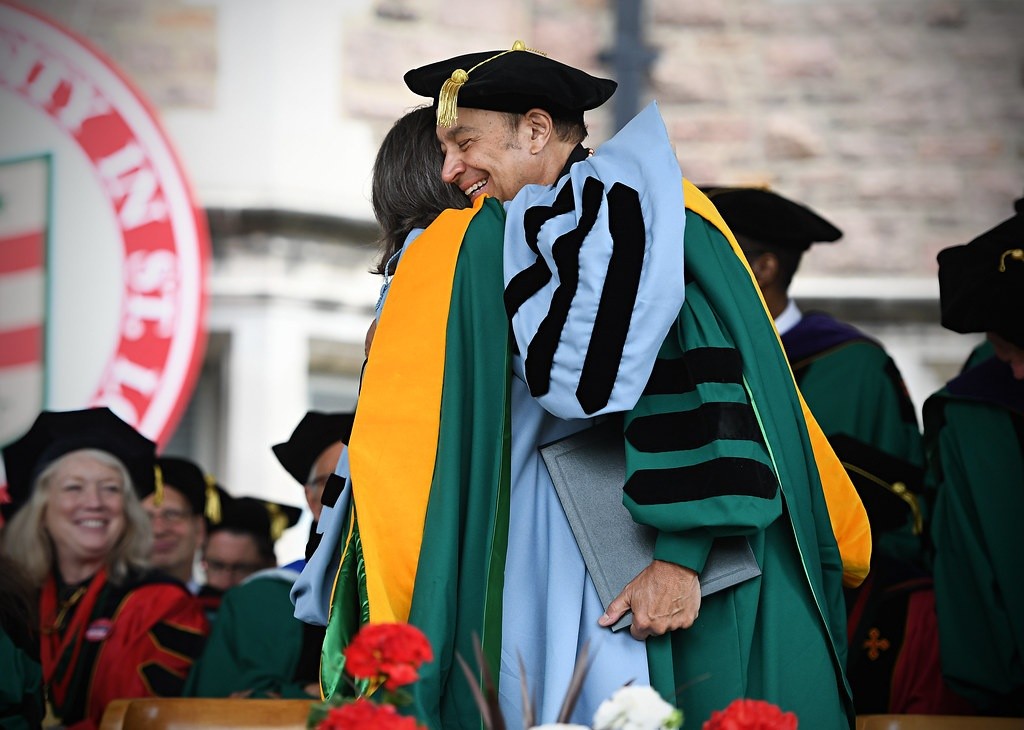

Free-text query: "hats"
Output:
<box><xmin>271</xmin><ymin>411</ymin><xmax>355</xmax><ymax>486</ymax></box>
<box><xmin>404</xmin><ymin>40</ymin><xmax>619</xmax><ymax>127</ymax></box>
<box><xmin>696</xmin><ymin>184</ymin><xmax>843</xmax><ymax>258</ymax></box>
<box><xmin>0</xmin><ymin>407</ymin><xmax>303</xmax><ymax>555</ymax></box>
<box><xmin>937</xmin><ymin>197</ymin><xmax>1024</xmax><ymax>335</ymax></box>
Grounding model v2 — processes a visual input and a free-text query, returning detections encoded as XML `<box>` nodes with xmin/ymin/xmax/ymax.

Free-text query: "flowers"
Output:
<box><xmin>318</xmin><ymin>621</ymin><xmax>797</xmax><ymax>730</ymax></box>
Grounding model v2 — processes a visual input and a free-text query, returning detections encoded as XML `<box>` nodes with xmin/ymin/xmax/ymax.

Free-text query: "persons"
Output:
<box><xmin>0</xmin><ymin>406</ymin><xmax>355</xmax><ymax>730</ymax></box>
<box><xmin>404</xmin><ymin>39</ymin><xmax>871</xmax><ymax>730</ymax></box>
<box><xmin>695</xmin><ymin>186</ymin><xmax>925</xmax><ymax>717</ymax></box>
<box><xmin>288</xmin><ymin>105</ymin><xmax>686</xmax><ymax>730</ymax></box>
<box><xmin>921</xmin><ymin>196</ymin><xmax>1024</xmax><ymax>717</ymax></box>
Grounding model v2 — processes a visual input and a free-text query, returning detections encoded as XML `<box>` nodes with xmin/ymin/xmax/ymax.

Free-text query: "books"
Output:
<box><xmin>537</xmin><ymin>418</ymin><xmax>762</xmax><ymax>633</ymax></box>
